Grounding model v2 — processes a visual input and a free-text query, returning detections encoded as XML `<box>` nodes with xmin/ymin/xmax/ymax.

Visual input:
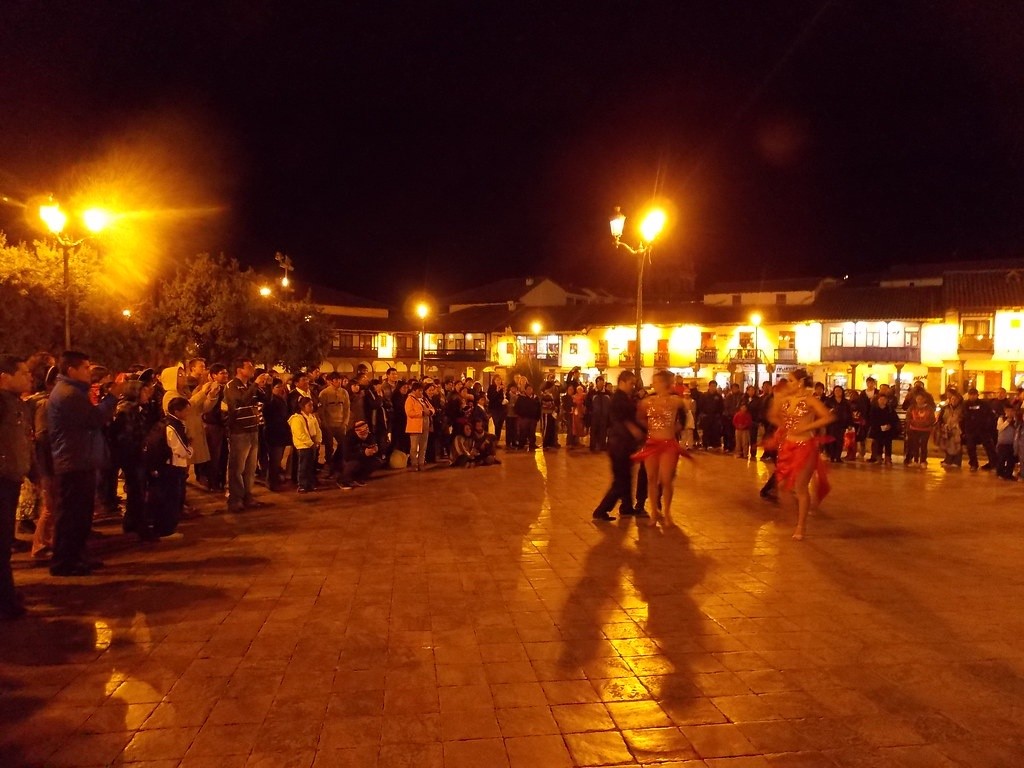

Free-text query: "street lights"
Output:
<box><xmin>412</xmin><ymin>297</ymin><xmax>434</xmax><ymax>383</ymax></box>
<box><xmin>609</xmin><ymin>203</ymin><xmax>666</xmax><ymax>381</ymax></box>
<box><xmin>747</xmin><ymin>311</ymin><xmax>760</xmax><ymax>393</ymax></box>
<box><xmin>33</xmin><ymin>199</ymin><xmax>116</xmax><ymax>349</ymax></box>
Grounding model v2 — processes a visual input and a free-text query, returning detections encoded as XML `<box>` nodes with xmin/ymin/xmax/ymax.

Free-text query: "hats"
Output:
<box><xmin>355</xmin><ymin>421</ymin><xmax>369</xmax><ymax>433</ymax></box>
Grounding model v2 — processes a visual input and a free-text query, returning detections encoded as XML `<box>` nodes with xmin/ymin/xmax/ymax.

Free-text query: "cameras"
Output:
<box><xmin>264</xmin><ymin>374</ymin><xmax>270</xmax><ymax>380</ymax></box>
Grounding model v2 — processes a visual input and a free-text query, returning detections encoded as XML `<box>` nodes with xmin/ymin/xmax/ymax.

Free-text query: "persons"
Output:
<box><xmin>0</xmin><ymin>353</ymin><xmax>1024</xmax><ymax>587</ymax></box>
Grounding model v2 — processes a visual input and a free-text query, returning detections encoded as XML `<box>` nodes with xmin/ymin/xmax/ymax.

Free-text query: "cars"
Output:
<box><xmin>935</xmin><ymin>392</ymin><xmax>1018</xmax><ymax>448</ymax></box>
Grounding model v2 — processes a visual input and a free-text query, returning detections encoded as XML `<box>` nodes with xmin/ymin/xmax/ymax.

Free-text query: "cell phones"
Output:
<box><xmin>577</xmin><ymin>367</ymin><xmax>581</xmax><ymax>370</ymax></box>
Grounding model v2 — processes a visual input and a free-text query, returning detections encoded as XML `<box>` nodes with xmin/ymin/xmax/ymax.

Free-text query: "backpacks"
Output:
<box><xmin>142</xmin><ymin>418</ymin><xmax>172</xmax><ymax>465</ymax></box>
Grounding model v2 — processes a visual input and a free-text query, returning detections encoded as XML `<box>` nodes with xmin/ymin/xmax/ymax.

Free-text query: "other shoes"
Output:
<box><xmin>245</xmin><ymin>500</ymin><xmax>264</xmax><ymax>507</ymax></box>
<box><xmin>354</xmin><ymin>480</ymin><xmax>368</xmax><ymax>487</ymax></box>
<box><xmin>160</xmin><ymin>532</ymin><xmax>184</xmax><ymax>540</ymax></box>
<box><xmin>297</xmin><ymin>486</ymin><xmax>306</xmax><ymax>494</ymax></box>
<box><xmin>30</xmin><ymin>547</ymin><xmax>54</xmax><ymax>561</ymax></box>
<box><xmin>636</xmin><ymin>506</ymin><xmax>650</xmax><ymax>517</ymax></box>
<box><xmin>229</xmin><ymin>503</ymin><xmax>245</xmax><ymax>513</ymax></box>
<box><xmin>619</xmin><ymin>504</ymin><xmax>635</xmax><ymax>514</ymax></box>
<box><xmin>593</xmin><ymin>509</ymin><xmax>616</xmax><ymax>521</ymax></box>
<box><xmin>337</xmin><ymin>480</ymin><xmax>352</xmax><ymax>490</ymax></box>
<box><xmin>49</xmin><ymin>560</ymin><xmax>92</xmax><ymax>576</ymax></box>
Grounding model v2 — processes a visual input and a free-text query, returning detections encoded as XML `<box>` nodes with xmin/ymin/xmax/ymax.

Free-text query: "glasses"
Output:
<box><xmin>216</xmin><ymin>371</ymin><xmax>229</xmax><ymax>376</ymax></box>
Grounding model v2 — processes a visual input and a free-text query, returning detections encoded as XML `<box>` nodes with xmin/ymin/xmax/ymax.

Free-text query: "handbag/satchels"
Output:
<box><xmin>389</xmin><ymin>449</ymin><xmax>408</xmax><ymax>469</ymax></box>
<box><xmin>15</xmin><ymin>477</ymin><xmax>40</xmax><ymax>521</ymax></box>
